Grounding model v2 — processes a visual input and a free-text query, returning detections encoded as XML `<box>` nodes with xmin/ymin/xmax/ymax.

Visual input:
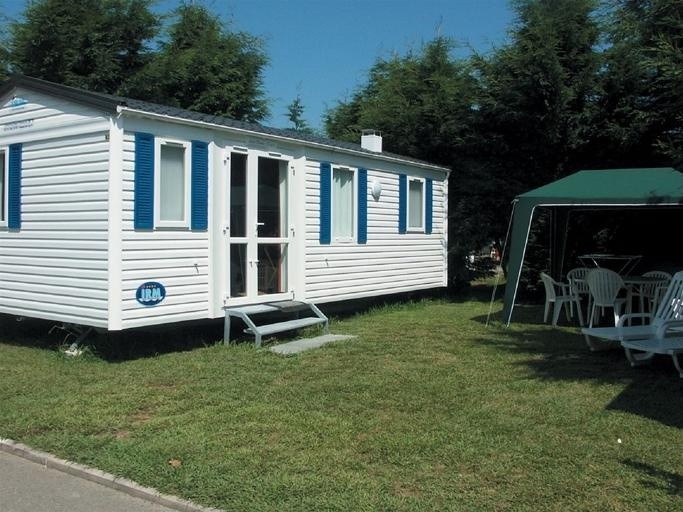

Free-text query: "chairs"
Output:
<box><xmin>538</xmin><ymin>255</ymin><xmax>683</xmax><ymax>378</ymax></box>
